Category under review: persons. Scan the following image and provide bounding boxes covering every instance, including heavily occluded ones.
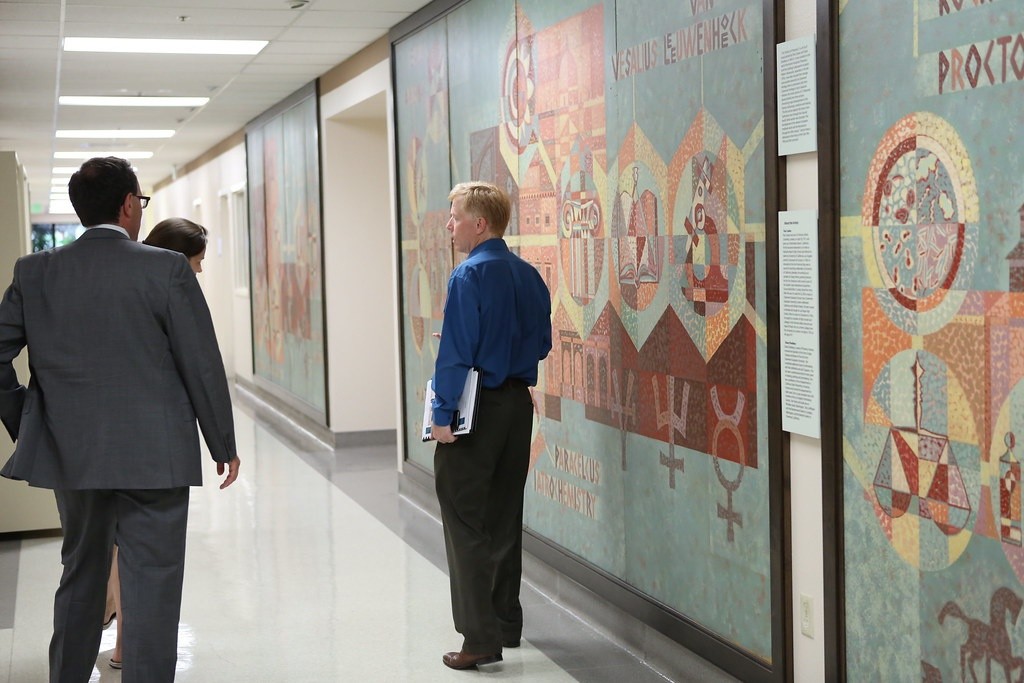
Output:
[431,180,553,668]
[0,156,241,683]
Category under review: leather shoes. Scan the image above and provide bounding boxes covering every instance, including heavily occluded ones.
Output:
[502,637,520,647]
[442,651,503,669]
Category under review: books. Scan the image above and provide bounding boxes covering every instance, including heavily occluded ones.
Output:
[422,367,484,442]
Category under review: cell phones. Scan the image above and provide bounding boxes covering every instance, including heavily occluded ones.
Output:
[450,410,460,432]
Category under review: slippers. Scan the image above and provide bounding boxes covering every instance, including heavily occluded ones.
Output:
[102,612,117,630]
[109,658,122,669]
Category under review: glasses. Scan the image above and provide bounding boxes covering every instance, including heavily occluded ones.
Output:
[121,196,150,208]
